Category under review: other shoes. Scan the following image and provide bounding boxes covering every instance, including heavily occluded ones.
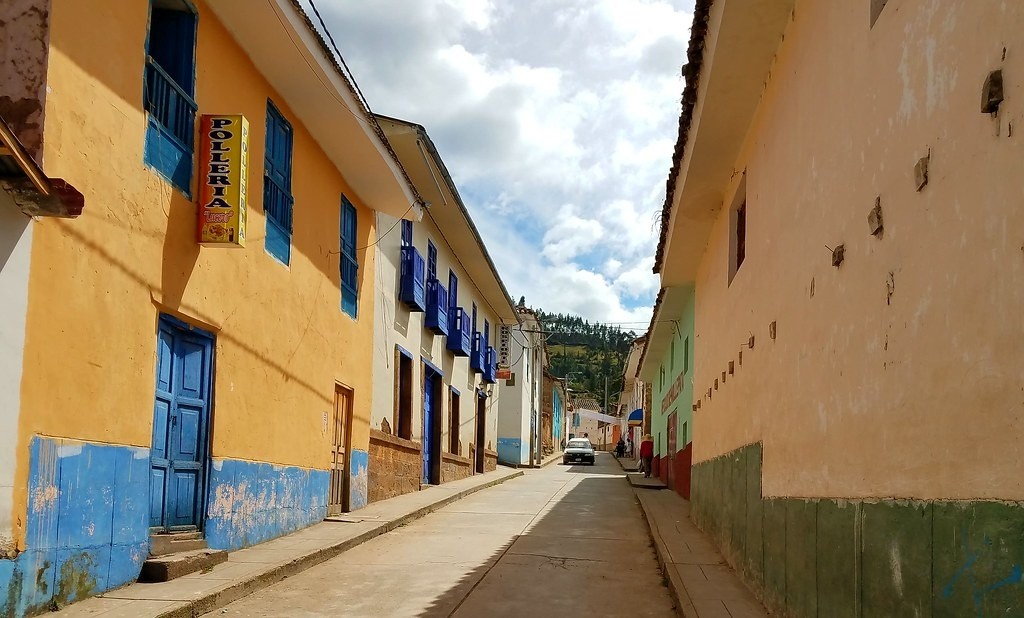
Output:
[619,457,621,458]
[644,473,650,478]
[623,456,624,458]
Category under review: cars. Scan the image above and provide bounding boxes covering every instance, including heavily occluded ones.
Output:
[562,438,596,466]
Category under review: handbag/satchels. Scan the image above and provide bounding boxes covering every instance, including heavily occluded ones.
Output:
[636,458,643,473]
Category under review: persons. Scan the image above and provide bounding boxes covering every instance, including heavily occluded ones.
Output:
[616,437,633,458]
[640,434,654,478]
[561,438,566,451]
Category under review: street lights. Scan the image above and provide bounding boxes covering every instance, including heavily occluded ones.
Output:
[564,371,583,448]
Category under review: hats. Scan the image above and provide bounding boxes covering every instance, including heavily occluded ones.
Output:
[641,434,652,441]
[584,433,588,436]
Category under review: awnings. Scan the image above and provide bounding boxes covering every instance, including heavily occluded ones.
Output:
[628,409,643,427]
